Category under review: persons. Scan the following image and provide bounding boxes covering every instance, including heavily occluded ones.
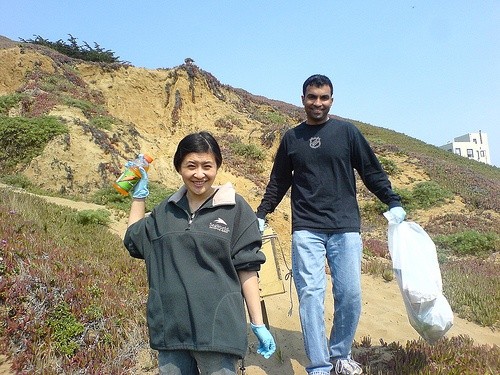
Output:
[124,131,275,375]
[254,74,407,375]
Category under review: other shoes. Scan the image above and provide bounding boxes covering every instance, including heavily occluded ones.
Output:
[330,357,363,375]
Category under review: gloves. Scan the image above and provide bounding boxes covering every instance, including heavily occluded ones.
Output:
[258,218,265,232]
[251,322,276,359]
[390,207,406,224]
[124,153,149,198]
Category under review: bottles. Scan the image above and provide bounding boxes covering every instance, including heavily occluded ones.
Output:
[113,154,154,196]
